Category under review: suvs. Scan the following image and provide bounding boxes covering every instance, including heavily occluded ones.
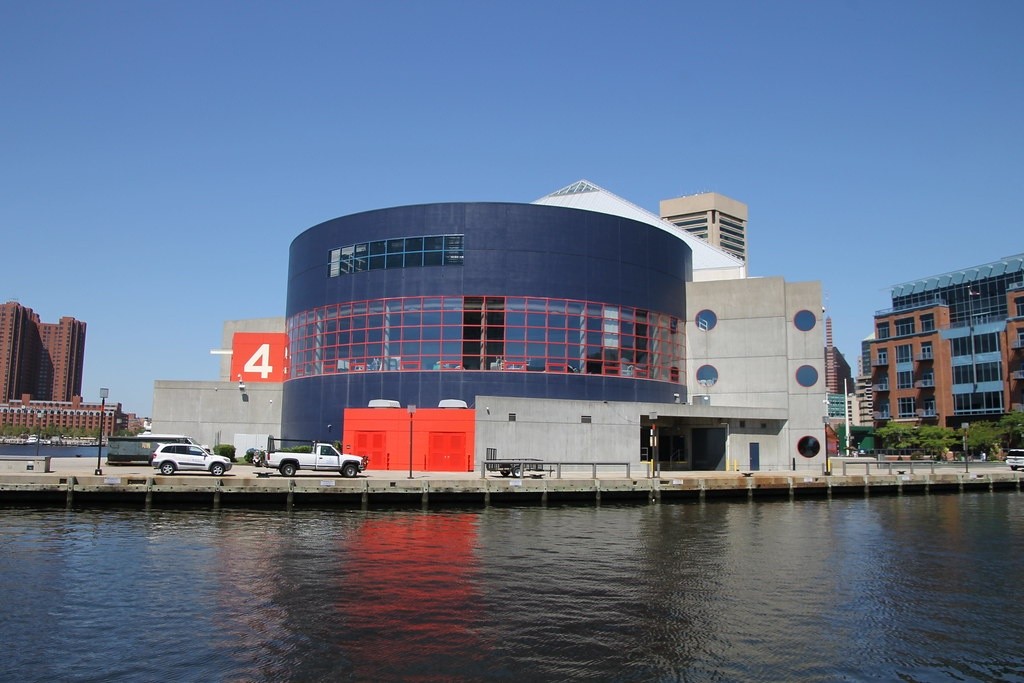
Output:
[1005,449,1024,470]
[151,442,233,476]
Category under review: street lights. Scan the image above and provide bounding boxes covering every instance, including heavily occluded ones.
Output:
[406,403,416,479]
[962,422,970,474]
[648,411,658,478]
[36,413,42,456]
[94,387,109,476]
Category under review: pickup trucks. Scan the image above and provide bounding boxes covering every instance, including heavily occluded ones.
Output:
[264,441,364,477]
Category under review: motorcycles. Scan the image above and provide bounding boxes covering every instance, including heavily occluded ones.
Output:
[252,448,262,467]
